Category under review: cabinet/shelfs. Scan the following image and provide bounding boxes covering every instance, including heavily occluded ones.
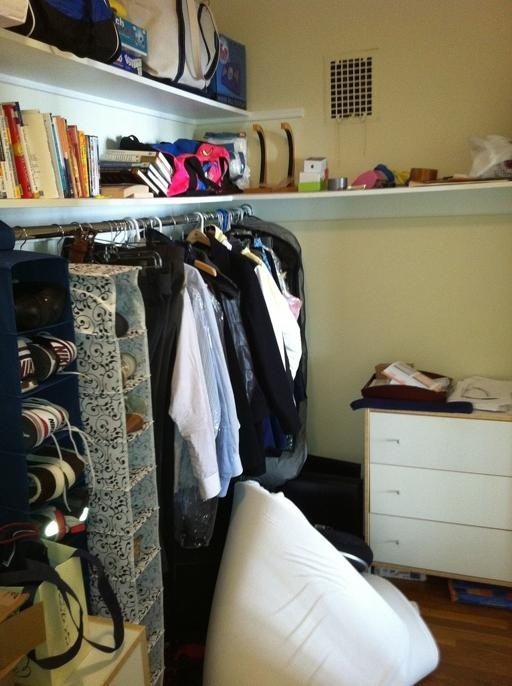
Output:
[66,260,167,686]
[0,218,94,611]
[361,406,512,595]
[0,23,247,209]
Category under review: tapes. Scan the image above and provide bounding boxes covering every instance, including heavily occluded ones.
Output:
[410,168,438,182]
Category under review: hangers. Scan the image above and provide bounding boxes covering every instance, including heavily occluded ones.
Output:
[13,204,251,275]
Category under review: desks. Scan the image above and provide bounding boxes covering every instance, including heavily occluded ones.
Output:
[57,613,152,686]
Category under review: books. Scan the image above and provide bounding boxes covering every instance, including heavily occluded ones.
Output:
[0,101,174,199]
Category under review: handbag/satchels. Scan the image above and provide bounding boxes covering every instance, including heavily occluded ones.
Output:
[1,542,126,684]
[121,135,243,196]
[17,0,122,66]
[122,0,222,94]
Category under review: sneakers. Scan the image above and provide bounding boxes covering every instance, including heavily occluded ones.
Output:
[117,314,146,435]
[14,273,89,542]
